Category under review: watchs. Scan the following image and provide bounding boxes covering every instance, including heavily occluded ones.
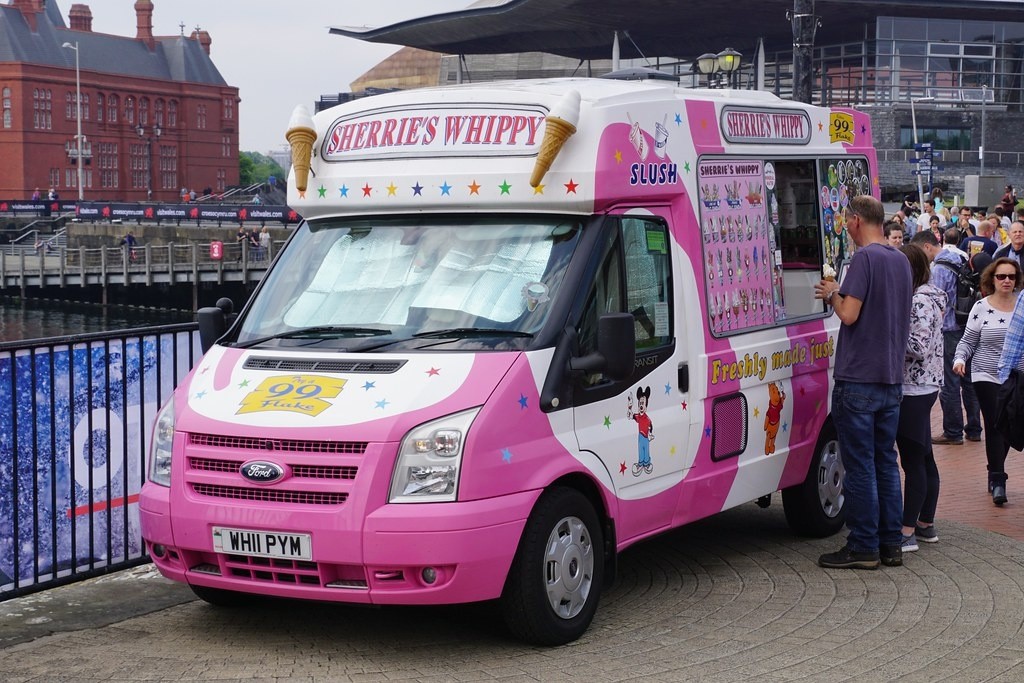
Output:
[827,289,840,304]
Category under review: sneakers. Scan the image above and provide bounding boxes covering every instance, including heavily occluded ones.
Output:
[878,546,903,566]
[900,533,919,553]
[913,524,938,542]
[819,541,881,569]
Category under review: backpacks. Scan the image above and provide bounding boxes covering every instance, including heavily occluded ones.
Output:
[935,260,978,325]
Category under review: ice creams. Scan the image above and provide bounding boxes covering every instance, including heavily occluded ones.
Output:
[530,89,582,188]
[285,104,318,190]
[704,214,772,331]
[823,267,837,282]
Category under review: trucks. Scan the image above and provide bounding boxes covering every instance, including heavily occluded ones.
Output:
[137,76,884,647]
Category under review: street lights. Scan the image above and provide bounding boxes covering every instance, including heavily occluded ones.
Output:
[697,48,719,89]
[717,47,744,89]
[61,41,94,199]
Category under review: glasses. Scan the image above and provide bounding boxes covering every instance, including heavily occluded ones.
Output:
[991,273,1017,281]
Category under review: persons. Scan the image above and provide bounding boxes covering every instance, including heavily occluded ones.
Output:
[814,196,912,569]
[953,258,1024,504]
[34,188,41,200]
[269,174,276,192]
[895,244,948,551]
[180,185,196,204]
[249,194,261,205]
[203,185,212,198]
[46,188,55,200]
[911,231,983,444]
[883,185,1024,382]
[237,226,270,263]
[34,238,44,253]
[119,231,137,265]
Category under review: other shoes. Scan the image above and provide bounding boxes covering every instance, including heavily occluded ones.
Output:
[929,433,963,445]
[965,432,981,441]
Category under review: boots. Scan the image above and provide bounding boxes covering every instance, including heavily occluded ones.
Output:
[985,465,1008,505]
